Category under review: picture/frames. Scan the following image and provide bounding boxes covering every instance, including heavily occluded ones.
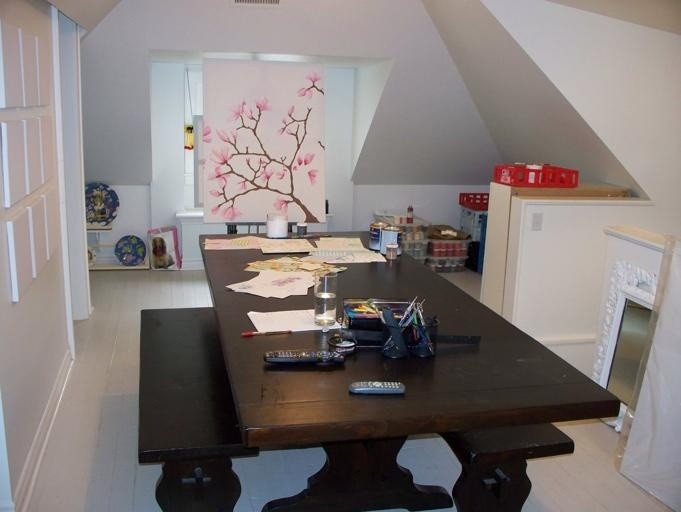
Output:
[596,286,655,434]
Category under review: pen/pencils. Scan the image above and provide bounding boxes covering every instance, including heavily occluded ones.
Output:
[345,295,435,355]
[241,330,292,337]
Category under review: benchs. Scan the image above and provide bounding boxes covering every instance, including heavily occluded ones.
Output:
[438,419,574,512]
[138,308,259,512]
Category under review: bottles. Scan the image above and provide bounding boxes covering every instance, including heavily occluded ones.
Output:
[297,222,308,236]
[385,243,399,261]
[407,205,413,224]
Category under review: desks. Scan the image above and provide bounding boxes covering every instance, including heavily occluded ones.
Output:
[198,230,620,512]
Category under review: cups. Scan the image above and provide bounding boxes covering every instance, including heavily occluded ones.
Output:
[265,205,288,239]
[524,164,543,186]
[314,269,337,327]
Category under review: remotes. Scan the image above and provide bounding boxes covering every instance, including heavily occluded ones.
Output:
[264,350,345,365]
[349,381,406,394]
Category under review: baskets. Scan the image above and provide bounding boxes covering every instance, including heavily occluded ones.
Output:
[492,161,581,189]
[458,190,490,210]
[373,211,489,273]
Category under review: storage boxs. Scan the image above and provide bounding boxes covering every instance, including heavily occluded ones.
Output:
[460,207,488,242]
